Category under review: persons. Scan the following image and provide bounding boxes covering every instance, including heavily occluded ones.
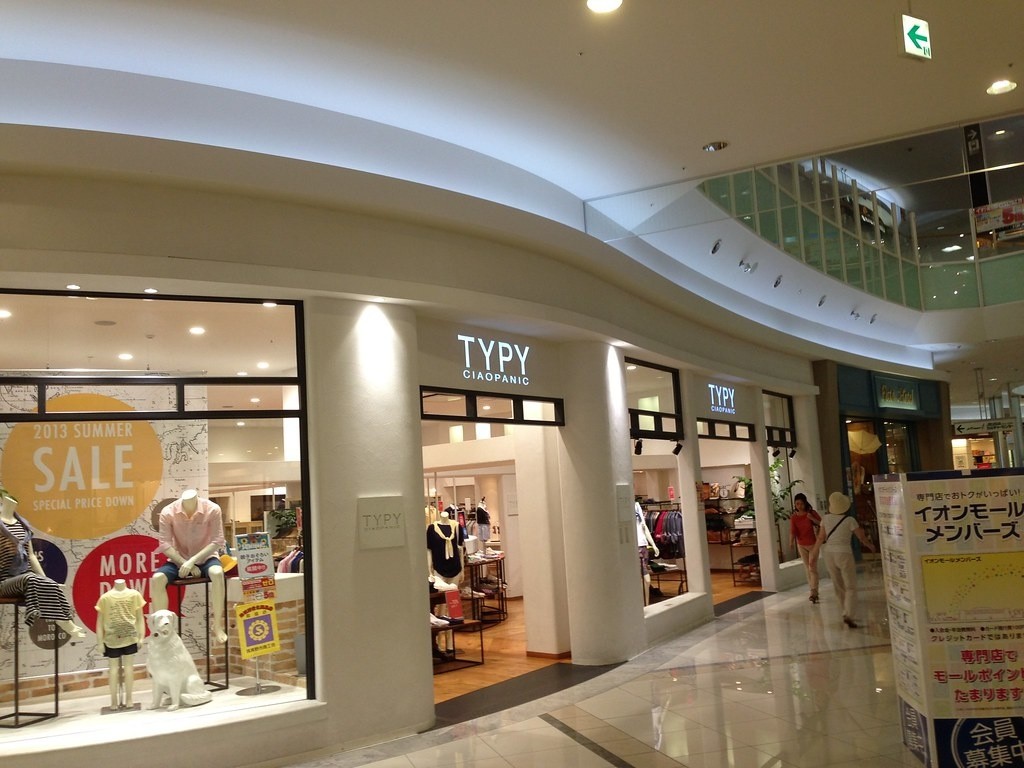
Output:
[477,501,491,554]
[808,492,876,628]
[635,501,660,606]
[94,579,147,710]
[0,496,87,642]
[790,493,821,604]
[152,489,228,643]
[426,512,464,654]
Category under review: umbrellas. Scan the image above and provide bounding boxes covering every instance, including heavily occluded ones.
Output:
[847,430,882,454]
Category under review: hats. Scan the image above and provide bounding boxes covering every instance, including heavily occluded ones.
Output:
[828,491,851,514]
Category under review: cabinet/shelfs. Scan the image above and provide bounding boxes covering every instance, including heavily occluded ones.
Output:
[704,498,748,545]
[455,560,509,633]
[730,545,761,587]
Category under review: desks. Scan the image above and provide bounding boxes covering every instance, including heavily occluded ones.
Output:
[169,574,230,693]
[0,597,59,728]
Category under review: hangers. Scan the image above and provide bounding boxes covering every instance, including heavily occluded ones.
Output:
[293,539,303,552]
[643,503,681,513]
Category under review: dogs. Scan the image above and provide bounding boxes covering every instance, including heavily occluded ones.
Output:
[143,609,213,711]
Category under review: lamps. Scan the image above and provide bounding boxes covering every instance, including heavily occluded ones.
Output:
[788,448,797,458]
[772,448,780,457]
[634,439,643,455]
[672,442,683,455]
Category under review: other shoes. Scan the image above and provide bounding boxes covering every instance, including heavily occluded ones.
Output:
[808,596,819,603]
[843,616,857,627]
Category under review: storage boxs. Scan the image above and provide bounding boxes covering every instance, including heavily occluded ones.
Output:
[734,519,754,529]
